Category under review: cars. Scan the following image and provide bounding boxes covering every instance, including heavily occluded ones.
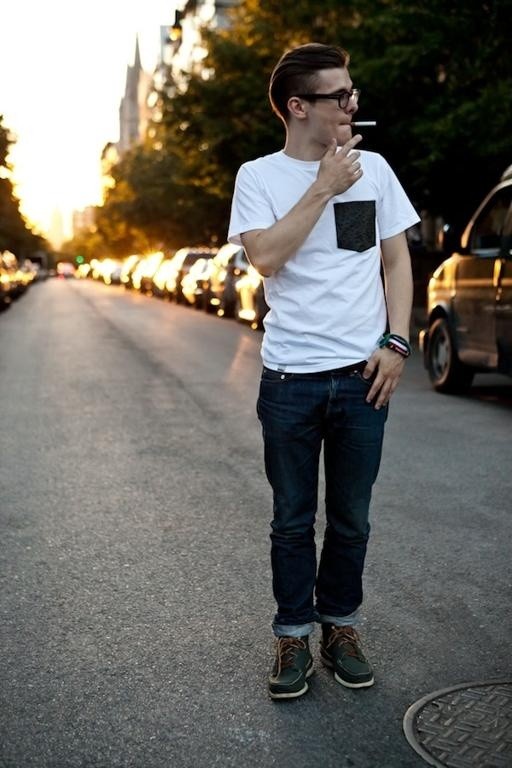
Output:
[84,244,270,329]
[0,251,39,313]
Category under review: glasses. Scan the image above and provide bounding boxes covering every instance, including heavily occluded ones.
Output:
[293,90,359,109]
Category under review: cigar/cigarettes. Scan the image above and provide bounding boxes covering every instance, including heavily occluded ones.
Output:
[347,121,378,127]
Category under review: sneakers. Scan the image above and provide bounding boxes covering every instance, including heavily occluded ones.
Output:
[320,623,374,688]
[268,637,315,698]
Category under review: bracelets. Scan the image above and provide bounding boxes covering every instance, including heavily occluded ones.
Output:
[380,333,412,359]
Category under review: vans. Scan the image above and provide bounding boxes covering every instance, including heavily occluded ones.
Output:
[419,165,512,391]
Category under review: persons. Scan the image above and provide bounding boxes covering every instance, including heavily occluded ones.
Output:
[227,42,421,702]
